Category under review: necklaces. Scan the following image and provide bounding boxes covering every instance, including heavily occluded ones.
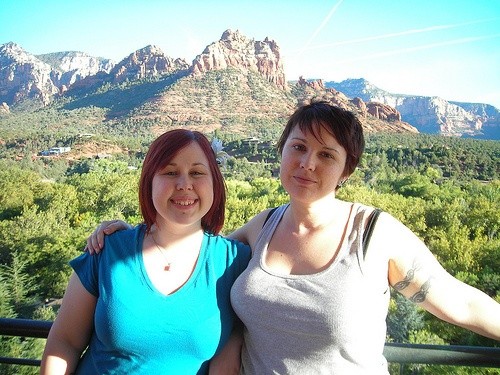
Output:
[150,233,172,271]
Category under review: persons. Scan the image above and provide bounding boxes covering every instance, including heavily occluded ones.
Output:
[82,99,500,375]
[41,129,252,375]
